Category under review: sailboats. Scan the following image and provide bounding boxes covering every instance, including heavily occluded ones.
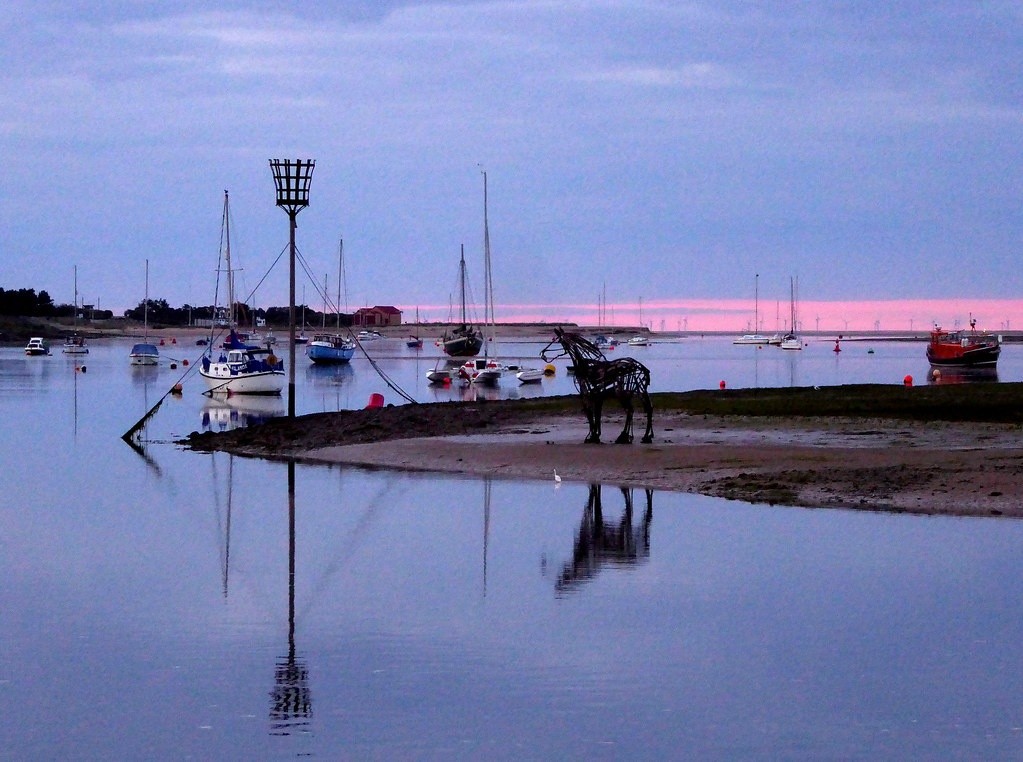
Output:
[406,306,423,348]
[442,243,484,356]
[129,258,158,366]
[591,281,650,349]
[199,189,286,394]
[733,274,803,350]
[63,265,88,353]
[295,239,356,363]
[425,171,502,384]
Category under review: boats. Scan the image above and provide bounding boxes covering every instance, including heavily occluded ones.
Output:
[435,342,444,347]
[516,365,545,382]
[565,360,637,379]
[925,312,1002,367]
[358,329,382,341]
[24,337,50,355]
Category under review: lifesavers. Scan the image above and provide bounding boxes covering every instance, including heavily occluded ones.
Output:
[466,337,475,346]
[267,354,277,365]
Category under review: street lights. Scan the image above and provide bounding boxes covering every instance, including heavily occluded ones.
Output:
[267,158,317,419]
